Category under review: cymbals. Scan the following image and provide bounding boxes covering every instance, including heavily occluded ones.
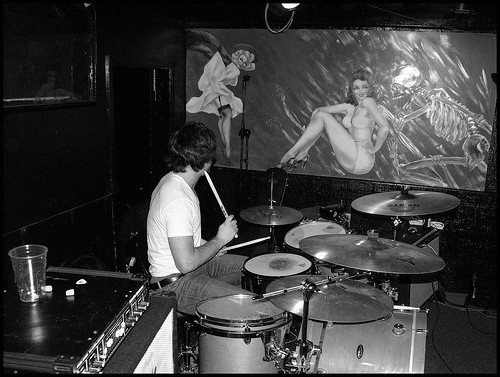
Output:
[266,273,394,327]
[239,205,304,226]
[351,187,462,216]
[298,232,446,275]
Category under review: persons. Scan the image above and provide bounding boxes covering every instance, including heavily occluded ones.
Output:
[149,120,256,314]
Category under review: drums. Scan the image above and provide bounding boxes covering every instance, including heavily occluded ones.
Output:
[241,252,314,295]
[295,309,428,374]
[194,291,294,374]
[283,219,347,259]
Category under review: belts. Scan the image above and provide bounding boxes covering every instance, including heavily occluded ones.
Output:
[149,274,182,290]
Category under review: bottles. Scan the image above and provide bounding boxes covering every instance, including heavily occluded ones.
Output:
[469,272,480,303]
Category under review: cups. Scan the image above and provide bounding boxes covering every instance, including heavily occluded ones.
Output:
[9,245,48,302]
